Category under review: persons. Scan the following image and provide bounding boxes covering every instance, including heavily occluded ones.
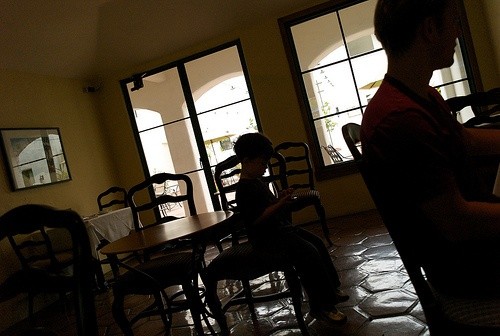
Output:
[234,134,350,326]
[362,0,500,336]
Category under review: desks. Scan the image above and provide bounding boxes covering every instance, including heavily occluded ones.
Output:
[99,209,235,336]
[32,207,143,293]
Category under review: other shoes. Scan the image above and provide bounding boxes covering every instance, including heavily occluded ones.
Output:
[309,307,348,324]
[329,292,350,305]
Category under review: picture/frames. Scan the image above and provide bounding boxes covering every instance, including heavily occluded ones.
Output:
[0,126,73,193]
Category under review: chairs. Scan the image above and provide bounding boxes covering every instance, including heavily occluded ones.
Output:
[201,151,312,336]
[0,204,98,336]
[445,87,500,130]
[7,226,101,322]
[95,186,127,285]
[267,140,334,248]
[342,123,500,336]
[128,172,207,327]
